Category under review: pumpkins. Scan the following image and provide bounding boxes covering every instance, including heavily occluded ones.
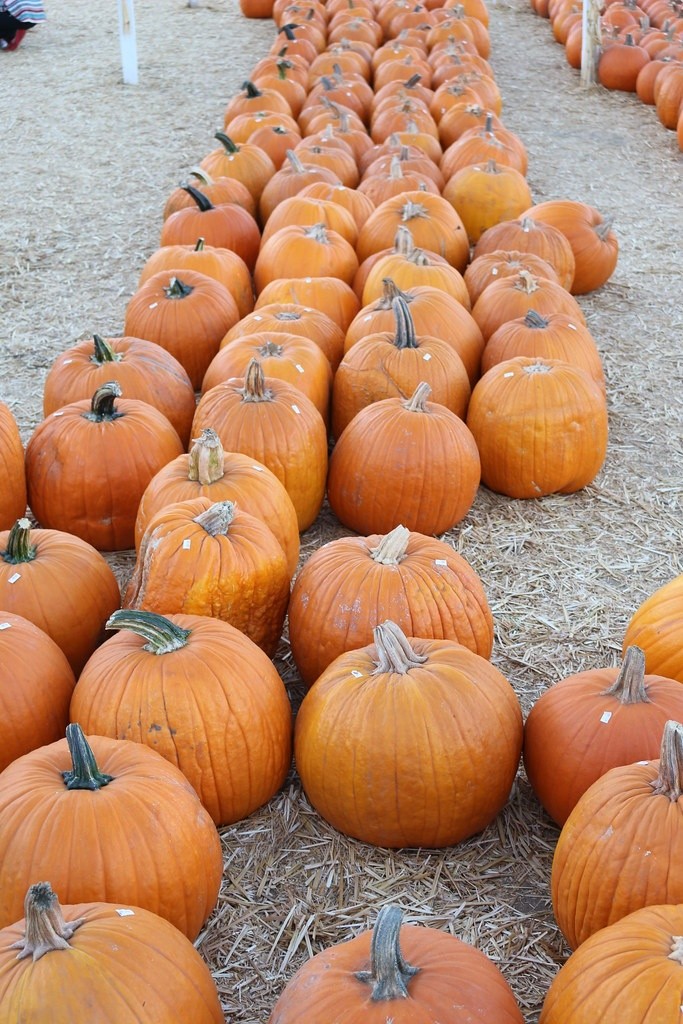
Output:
[0,0,683,1024]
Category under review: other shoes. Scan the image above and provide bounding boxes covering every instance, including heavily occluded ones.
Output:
[5,28,27,51]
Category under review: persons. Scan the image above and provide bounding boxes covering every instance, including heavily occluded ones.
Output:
[0,0,49,50]
